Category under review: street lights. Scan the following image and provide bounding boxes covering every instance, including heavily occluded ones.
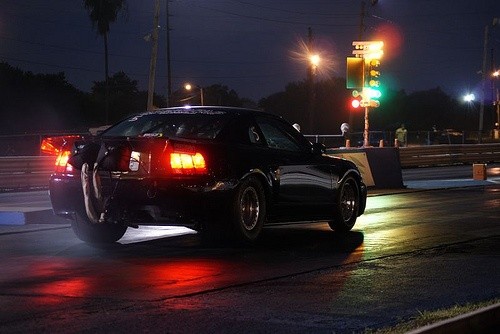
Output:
[305,27,322,144]
[186,84,204,106]
[351,41,383,147]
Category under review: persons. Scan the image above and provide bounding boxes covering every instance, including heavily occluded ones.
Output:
[429,125,439,143]
[395,124,408,147]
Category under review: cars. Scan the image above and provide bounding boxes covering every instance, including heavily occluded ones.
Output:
[48,105,368,249]
[441,128,463,139]
